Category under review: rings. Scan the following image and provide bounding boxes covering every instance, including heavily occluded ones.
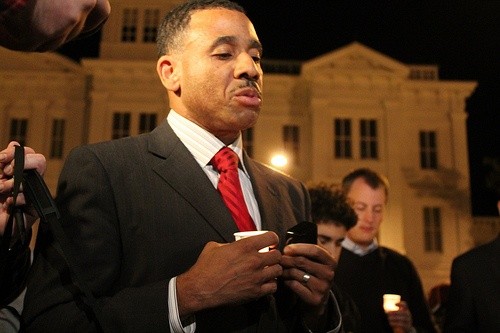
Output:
[300,272,311,284]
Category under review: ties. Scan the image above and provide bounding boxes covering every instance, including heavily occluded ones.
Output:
[207,147,257,232]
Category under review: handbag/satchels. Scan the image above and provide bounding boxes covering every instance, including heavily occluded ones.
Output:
[0,146,32,308]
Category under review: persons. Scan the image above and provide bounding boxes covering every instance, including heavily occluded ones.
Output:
[0,0,111,52]
[307,168,500,333]
[19,0,341,333]
[0,141,47,309]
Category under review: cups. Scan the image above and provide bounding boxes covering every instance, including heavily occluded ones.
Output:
[233,229,271,284]
[382,291,401,313]
